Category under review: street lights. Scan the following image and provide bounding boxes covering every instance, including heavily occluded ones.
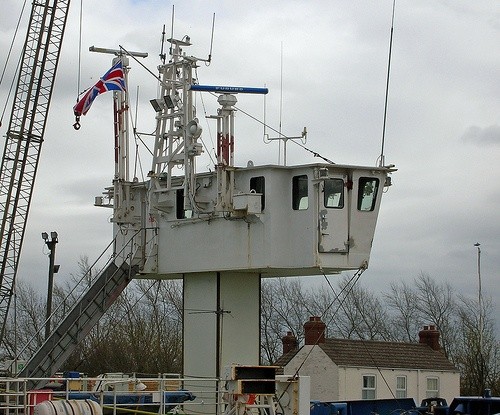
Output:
[41,231,60,340]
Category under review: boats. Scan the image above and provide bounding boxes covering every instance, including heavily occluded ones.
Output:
[0,0,500,415]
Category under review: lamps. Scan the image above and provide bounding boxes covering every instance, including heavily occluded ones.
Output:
[150,98,164,112]
[164,93,181,109]
[136,380,147,391]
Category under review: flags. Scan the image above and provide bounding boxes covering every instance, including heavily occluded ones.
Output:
[73,59,126,116]
